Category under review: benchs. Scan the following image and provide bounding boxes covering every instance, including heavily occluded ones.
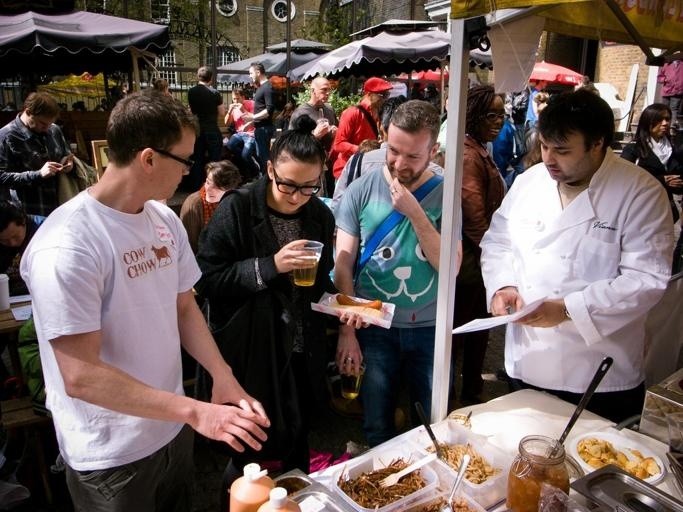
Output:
[1,372,201,503]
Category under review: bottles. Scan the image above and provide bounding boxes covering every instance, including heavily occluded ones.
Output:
[255,486,300,511]
[229,462,274,511]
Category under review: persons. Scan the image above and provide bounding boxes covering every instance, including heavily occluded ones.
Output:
[192,131,370,480]
[447,85,507,412]
[0,60,683,295]
[20,93,270,511]
[480,86,675,427]
[336,101,462,447]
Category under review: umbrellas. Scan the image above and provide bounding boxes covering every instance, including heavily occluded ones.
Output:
[210,37,333,87]
[286,18,493,115]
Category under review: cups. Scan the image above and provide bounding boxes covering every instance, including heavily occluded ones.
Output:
[292,240,325,288]
[338,360,367,400]
[0,274,11,311]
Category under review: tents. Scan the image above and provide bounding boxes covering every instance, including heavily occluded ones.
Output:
[0,11,177,93]
[432,0,683,421]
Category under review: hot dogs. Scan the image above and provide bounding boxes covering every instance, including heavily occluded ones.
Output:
[329,294,384,320]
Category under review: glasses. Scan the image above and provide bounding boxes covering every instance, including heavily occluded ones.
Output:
[273,167,321,196]
[378,93,390,97]
[487,112,510,120]
[152,148,194,171]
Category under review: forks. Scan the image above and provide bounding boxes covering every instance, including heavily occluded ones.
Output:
[377,451,437,489]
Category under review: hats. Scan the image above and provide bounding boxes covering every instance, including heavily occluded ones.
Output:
[364,77,393,92]
